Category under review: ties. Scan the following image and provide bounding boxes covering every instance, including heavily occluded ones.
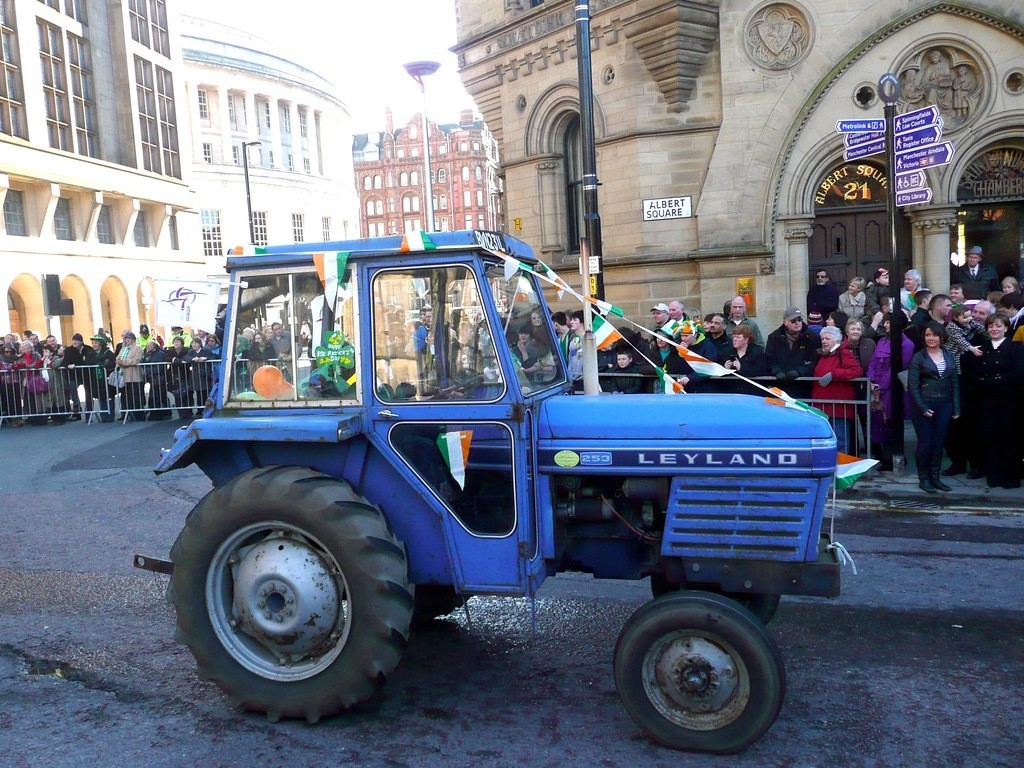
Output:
[972,269,975,277]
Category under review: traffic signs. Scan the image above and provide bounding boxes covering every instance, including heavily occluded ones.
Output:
[894,124,942,153]
[894,140,955,175]
[895,186,933,206]
[893,105,944,136]
[835,118,887,133]
[843,136,886,163]
[894,168,927,194]
[842,130,886,149]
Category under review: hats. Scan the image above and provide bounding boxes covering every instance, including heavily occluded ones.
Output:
[874,269,889,279]
[650,302,669,314]
[653,318,680,340]
[966,246,985,258]
[124,333,135,340]
[783,307,804,322]
[72,333,83,341]
[807,313,822,326]
[90,334,106,344]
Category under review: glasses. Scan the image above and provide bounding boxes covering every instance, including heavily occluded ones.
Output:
[4,350,13,354]
[787,317,804,324]
[815,274,826,279]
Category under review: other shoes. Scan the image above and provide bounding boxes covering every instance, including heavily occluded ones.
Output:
[878,460,909,471]
[967,469,979,478]
[12,411,204,427]
[942,460,967,475]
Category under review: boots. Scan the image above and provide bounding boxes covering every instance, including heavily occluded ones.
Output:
[931,449,951,492]
[916,452,938,493]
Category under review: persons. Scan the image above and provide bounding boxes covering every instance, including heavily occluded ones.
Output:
[232,322,303,397]
[0,322,221,428]
[950,246,1001,300]
[765,267,1024,494]
[597,293,768,397]
[376,302,584,402]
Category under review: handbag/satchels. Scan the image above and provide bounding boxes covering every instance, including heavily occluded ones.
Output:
[108,371,125,388]
[28,373,49,394]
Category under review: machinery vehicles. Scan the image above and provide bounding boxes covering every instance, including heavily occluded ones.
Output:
[133,227,847,757]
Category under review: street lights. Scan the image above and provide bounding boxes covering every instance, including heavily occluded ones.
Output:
[405,59,444,232]
[243,141,263,334]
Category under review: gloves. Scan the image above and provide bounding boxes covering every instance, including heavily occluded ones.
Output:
[819,372,835,388]
[775,372,789,389]
[789,369,799,379]
